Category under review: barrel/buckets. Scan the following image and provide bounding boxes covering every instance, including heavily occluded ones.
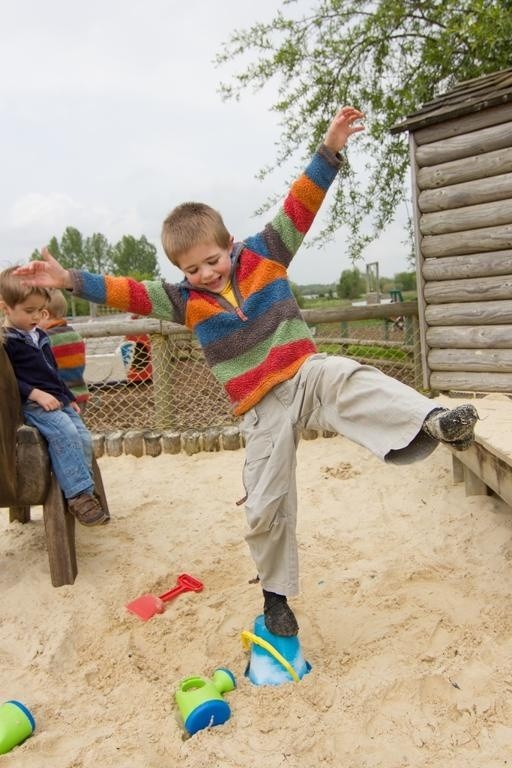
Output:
[241,613,312,686]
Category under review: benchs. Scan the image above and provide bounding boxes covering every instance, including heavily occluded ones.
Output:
[449,393,512,510]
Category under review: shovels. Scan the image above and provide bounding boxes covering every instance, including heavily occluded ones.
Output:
[126,574,203,622]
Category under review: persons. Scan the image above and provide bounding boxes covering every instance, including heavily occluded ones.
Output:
[38,286,89,417]
[0,267,109,528]
[12,107,477,639]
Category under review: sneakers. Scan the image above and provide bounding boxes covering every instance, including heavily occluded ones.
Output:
[68,492,106,526]
